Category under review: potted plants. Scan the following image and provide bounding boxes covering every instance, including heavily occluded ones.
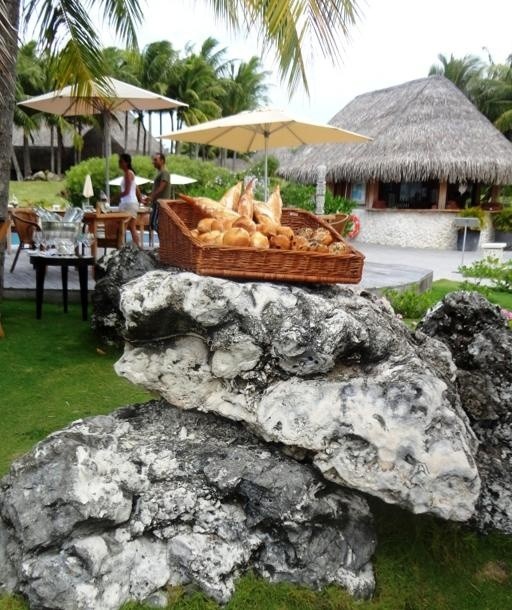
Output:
[454,204,512,251]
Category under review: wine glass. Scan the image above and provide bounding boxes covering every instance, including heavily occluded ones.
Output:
[33,231,95,259]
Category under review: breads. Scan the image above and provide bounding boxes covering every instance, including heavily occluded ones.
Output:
[176,179,352,254]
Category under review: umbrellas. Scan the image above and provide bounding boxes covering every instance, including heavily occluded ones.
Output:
[12,73,189,203]
[159,105,375,198]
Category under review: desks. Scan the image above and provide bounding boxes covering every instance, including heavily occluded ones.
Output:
[51,207,155,281]
[481,242,507,261]
[27,250,95,322]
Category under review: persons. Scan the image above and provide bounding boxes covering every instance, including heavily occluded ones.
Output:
[147,153,171,249]
[118,153,142,249]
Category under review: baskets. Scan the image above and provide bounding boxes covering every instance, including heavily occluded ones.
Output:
[157,196,362,284]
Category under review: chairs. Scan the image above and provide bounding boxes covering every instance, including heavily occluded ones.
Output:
[8,207,42,274]
[324,213,348,236]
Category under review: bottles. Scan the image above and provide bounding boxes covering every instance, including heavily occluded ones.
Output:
[100,191,107,206]
